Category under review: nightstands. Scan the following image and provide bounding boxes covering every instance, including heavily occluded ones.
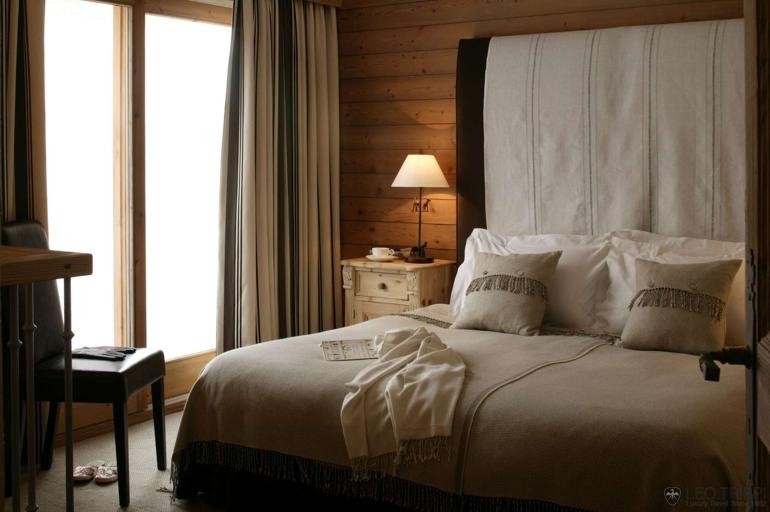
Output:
[339,256,457,327]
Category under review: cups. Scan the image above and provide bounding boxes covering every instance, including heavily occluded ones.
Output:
[372,248,394,257]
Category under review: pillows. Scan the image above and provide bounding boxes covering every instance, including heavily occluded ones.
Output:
[607,229,746,348]
[447,251,564,335]
[613,258,743,355]
[451,228,612,336]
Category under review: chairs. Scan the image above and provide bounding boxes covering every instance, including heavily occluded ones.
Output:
[3,218,166,510]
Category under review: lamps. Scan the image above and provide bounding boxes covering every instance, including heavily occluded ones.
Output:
[391,153,450,264]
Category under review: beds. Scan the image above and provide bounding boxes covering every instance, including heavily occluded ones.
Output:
[170,16,748,512]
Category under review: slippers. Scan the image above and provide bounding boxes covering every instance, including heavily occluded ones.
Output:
[72,461,118,483]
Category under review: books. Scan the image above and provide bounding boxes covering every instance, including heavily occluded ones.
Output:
[318,339,379,362]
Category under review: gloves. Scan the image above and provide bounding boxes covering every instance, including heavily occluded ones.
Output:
[72,345,137,361]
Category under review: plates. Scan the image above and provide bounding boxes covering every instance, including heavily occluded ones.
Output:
[366,255,394,262]
